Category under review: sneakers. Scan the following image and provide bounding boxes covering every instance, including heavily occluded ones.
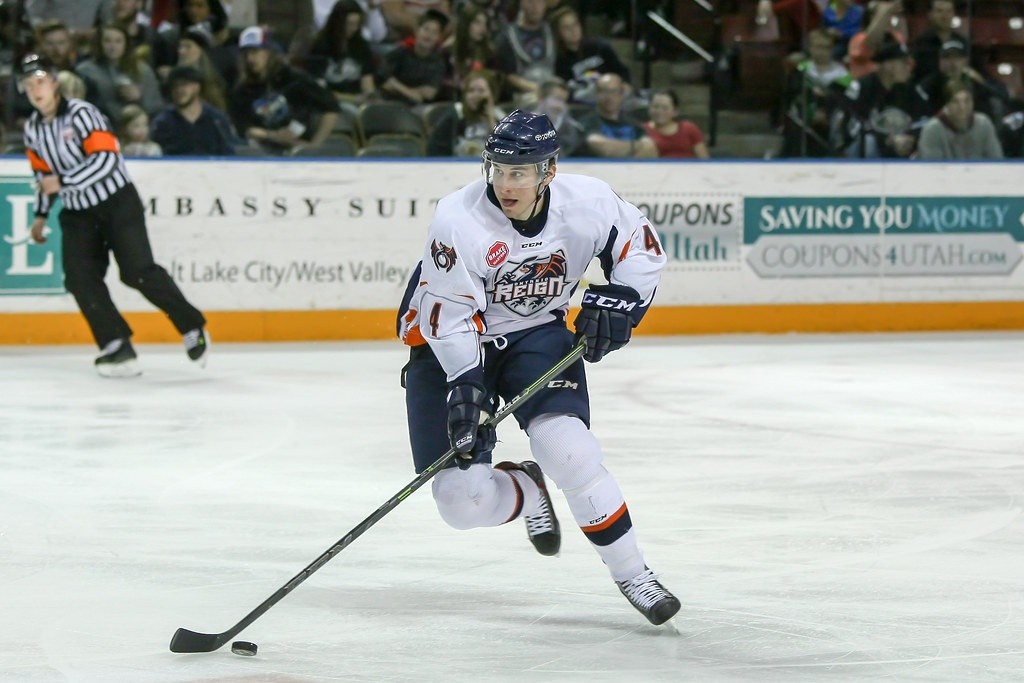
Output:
[494,461,561,558]
[95,337,142,378]
[602,558,681,636]
[184,329,210,369]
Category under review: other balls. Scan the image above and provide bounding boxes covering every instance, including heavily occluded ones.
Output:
[231,640,258,657]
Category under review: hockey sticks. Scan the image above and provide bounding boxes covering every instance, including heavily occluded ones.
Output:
[166,342,591,656]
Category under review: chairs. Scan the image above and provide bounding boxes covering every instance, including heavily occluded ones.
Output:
[286,101,457,162]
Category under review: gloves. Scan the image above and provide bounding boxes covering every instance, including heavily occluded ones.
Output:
[447,381,496,470]
[573,284,640,363]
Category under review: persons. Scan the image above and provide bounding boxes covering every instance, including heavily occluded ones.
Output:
[719,0,1024,158]
[0,0,713,159]
[15,55,212,378]
[396,110,684,635]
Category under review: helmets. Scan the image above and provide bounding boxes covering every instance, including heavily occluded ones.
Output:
[17,53,52,81]
[485,110,559,165]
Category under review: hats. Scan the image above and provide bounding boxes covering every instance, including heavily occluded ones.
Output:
[939,40,967,58]
[239,26,272,52]
[168,66,202,81]
[869,41,911,62]
[180,29,212,55]
